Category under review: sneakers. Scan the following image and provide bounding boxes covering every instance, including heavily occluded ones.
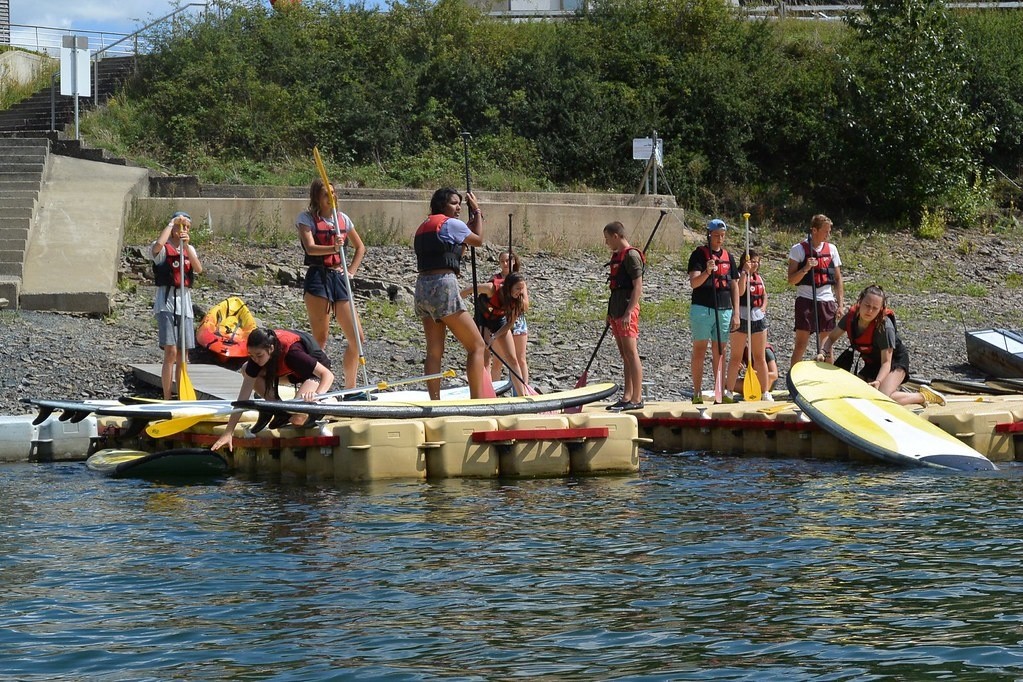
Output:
[725,390,733,399]
[692,396,703,403]
[919,384,947,409]
[761,391,774,402]
[606,399,644,410]
[714,396,734,403]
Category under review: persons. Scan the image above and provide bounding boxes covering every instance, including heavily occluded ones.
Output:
[788,215,845,396]
[725,251,774,401]
[603,221,646,410]
[726,335,779,394]
[294,178,378,401]
[810,284,947,407]
[414,188,485,401]
[458,272,524,398]
[489,252,529,396]
[686,218,740,405]
[210,327,335,452]
[150,212,202,400]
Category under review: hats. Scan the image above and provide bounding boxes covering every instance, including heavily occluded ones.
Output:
[172,212,192,222]
[708,219,726,231]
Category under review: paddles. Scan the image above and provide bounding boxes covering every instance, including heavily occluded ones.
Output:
[146,369,456,438]
[177,222,196,400]
[706,227,723,404]
[562,209,668,413]
[807,205,820,355]
[403,286,539,396]
[756,397,995,414]
[508,213,517,397]
[459,131,496,398]
[743,213,762,402]
[312,146,371,402]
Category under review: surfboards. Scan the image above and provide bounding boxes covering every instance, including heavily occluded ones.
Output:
[787,358,1002,474]
[84,447,229,477]
[18,379,515,427]
[231,380,619,417]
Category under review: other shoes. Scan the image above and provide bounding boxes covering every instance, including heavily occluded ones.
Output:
[269,412,292,429]
[303,413,325,429]
[343,392,378,401]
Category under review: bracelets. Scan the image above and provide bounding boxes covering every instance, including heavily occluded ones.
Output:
[332,246,336,254]
[472,207,483,217]
[800,267,808,274]
[819,349,828,357]
[705,269,710,276]
